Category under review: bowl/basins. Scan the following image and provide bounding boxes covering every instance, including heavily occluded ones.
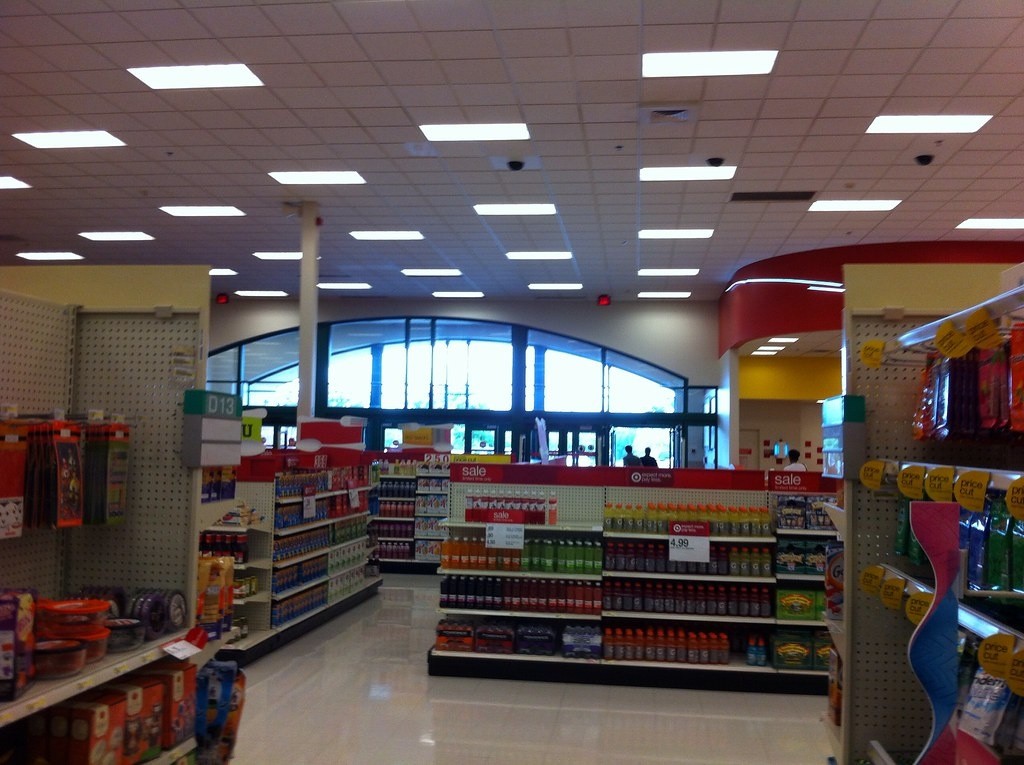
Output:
[30,597,145,679]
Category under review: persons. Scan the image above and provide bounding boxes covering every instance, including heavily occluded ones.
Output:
[783,449,808,472]
[639,447,658,466]
[623,446,643,467]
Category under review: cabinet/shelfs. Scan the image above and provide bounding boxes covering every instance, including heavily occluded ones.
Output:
[426,465,839,695]
[819,283,1024,765]
[202,464,390,671]
[372,456,453,576]
[0,260,213,765]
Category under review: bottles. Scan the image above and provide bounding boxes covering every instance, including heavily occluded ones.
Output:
[370,458,379,485]
[746,638,767,665]
[438,576,601,616]
[464,489,546,526]
[379,523,413,538]
[227,616,248,645]
[380,459,383,475]
[378,501,414,518]
[549,491,557,526]
[602,625,730,666]
[436,619,600,660]
[394,459,416,476]
[197,533,249,565]
[440,534,602,575]
[384,458,389,475]
[604,581,773,619]
[602,500,771,539]
[603,541,772,578]
[383,482,416,498]
[233,575,257,599]
[375,542,410,559]
[377,481,381,497]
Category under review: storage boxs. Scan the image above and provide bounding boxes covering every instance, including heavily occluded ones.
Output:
[827,547,843,621]
[828,647,843,727]
[22,661,201,765]
[412,477,448,561]
